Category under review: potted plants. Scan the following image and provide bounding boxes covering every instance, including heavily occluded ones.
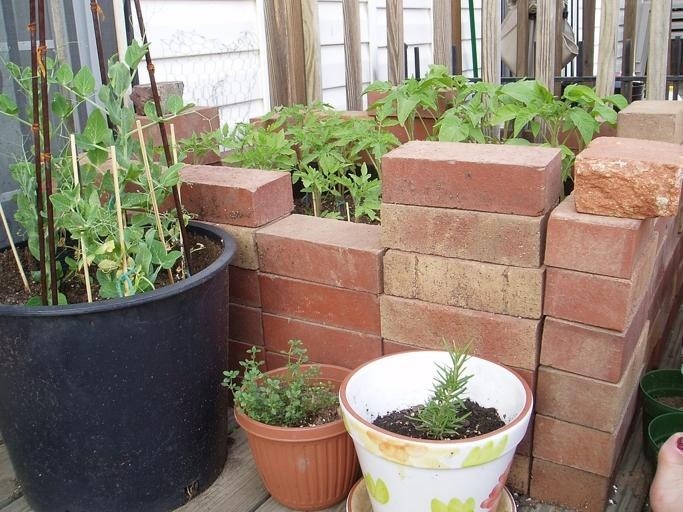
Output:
[0,16,238,512]
